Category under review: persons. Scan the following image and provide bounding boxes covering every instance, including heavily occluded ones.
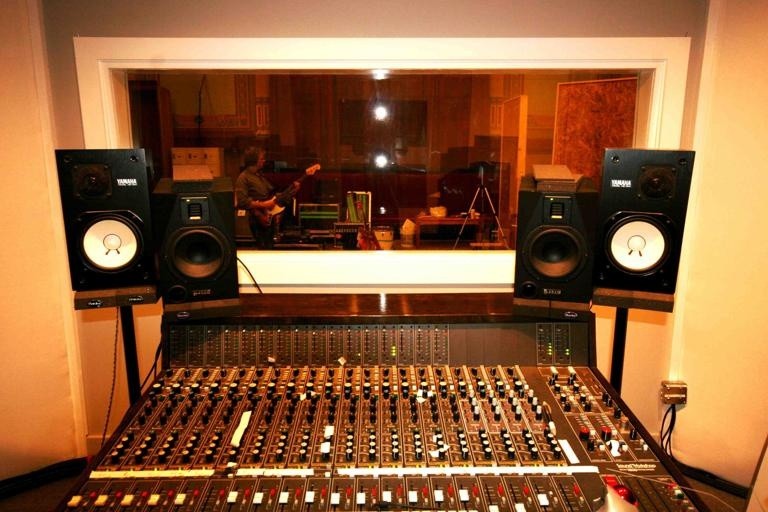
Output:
[234,145,300,250]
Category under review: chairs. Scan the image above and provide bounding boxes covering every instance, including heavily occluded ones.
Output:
[333,190,372,251]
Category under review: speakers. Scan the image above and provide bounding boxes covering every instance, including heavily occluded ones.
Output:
[150,177,240,304]
[512,176,601,304]
[54,148,153,292]
[595,147,695,295]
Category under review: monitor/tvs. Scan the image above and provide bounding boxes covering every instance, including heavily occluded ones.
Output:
[337,98,428,147]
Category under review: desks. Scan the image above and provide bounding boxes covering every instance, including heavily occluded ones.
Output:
[416,213,493,245]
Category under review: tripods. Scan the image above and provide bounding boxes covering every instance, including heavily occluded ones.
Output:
[452,168,509,250]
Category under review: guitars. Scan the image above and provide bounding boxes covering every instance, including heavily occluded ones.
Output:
[252,163,321,226]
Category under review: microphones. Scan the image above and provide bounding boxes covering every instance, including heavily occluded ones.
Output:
[264,160,287,170]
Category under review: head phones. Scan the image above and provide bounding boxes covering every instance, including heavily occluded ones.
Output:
[244,147,258,166]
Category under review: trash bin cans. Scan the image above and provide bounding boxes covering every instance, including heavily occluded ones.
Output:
[400,219,416,248]
